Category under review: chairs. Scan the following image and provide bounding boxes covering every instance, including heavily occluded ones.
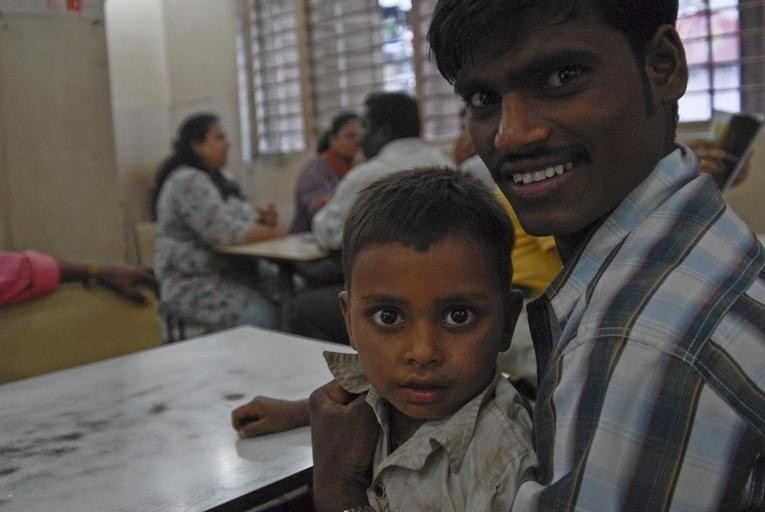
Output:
[133,220,189,343]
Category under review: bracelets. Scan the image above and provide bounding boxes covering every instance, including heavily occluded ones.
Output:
[79,260,98,291]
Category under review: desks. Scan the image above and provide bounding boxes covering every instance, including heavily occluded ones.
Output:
[217,233,344,334]
[0,323,368,512]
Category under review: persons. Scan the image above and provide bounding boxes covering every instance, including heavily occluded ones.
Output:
[146,111,282,335]
[231,167,543,512]
[293,88,563,297]
[308,0,764,512]
[0,250,159,308]
